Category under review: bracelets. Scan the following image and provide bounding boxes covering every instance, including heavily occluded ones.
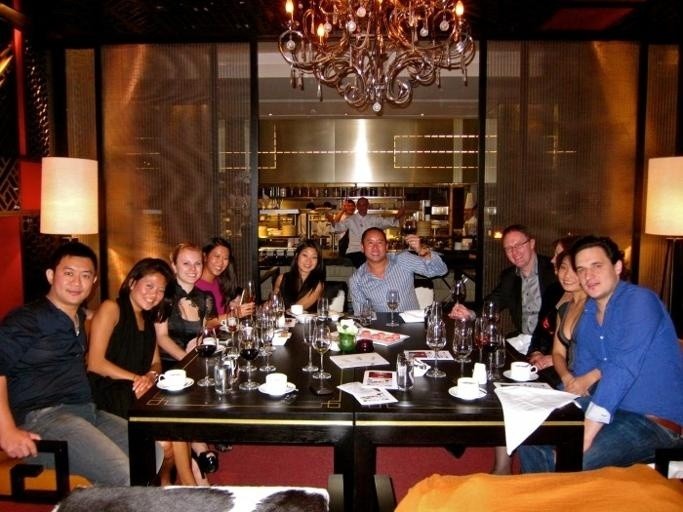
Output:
[419,251,429,257]
[131,373,137,381]
[144,371,159,383]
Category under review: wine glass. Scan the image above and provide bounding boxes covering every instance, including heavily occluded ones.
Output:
[196,279,507,394]
[402,215,418,254]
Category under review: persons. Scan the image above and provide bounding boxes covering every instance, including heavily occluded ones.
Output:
[444,224,558,460]
[153,241,218,486]
[490,237,579,477]
[348,227,448,316]
[335,200,355,251]
[555,250,592,400]
[0,244,164,486]
[194,237,255,452]
[519,236,682,472]
[273,240,325,311]
[85,256,198,485]
[325,198,406,259]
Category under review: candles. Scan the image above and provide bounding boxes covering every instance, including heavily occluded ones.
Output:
[455,1,464,24]
[317,23,324,44]
[286,1,294,20]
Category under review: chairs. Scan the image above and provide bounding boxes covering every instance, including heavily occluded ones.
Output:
[324,280,348,312]
[1,438,93,506]
[646,338,682,478]
[414,279,435,306]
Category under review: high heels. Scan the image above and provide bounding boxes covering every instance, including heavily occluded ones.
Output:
[197,451,218,478]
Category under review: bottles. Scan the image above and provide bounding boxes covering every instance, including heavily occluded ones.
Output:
[260,186,402,198]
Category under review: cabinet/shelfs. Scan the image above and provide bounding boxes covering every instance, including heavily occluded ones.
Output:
[258,186,451,249]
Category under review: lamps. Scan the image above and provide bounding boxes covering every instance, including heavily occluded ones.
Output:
[646,157,683,312]
[277,0,476,113]
[39,157,100,241]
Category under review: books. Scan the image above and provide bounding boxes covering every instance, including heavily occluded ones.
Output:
[362,369,399,391]
[329,352,390,369]
[404,350,455,362]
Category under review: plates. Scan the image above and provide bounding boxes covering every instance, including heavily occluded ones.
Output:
[259,224,298,238]
[155,304,540,400]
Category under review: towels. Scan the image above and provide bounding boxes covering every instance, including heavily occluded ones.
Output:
[493,386,583,458]
[398,309,427,324]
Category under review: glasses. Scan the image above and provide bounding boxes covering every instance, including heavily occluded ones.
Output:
[504,239,530,251]
